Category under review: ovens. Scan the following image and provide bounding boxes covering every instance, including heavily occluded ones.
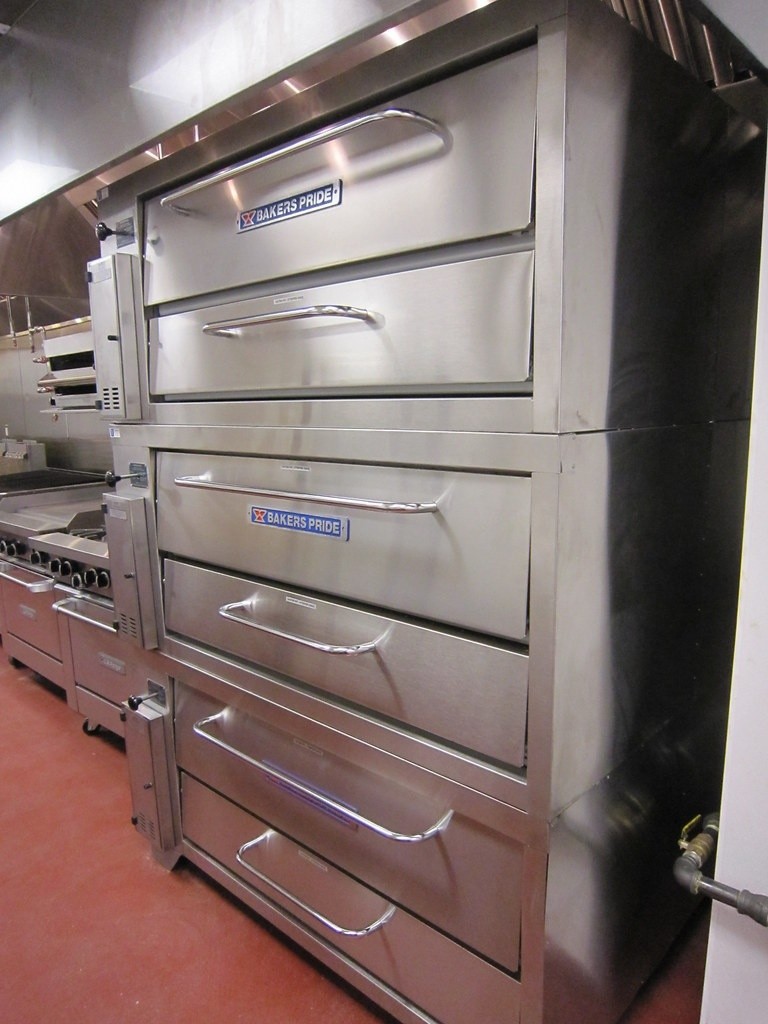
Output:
[86,0,768,1024]
[39,330,96,417]
[0,514,128,739]
